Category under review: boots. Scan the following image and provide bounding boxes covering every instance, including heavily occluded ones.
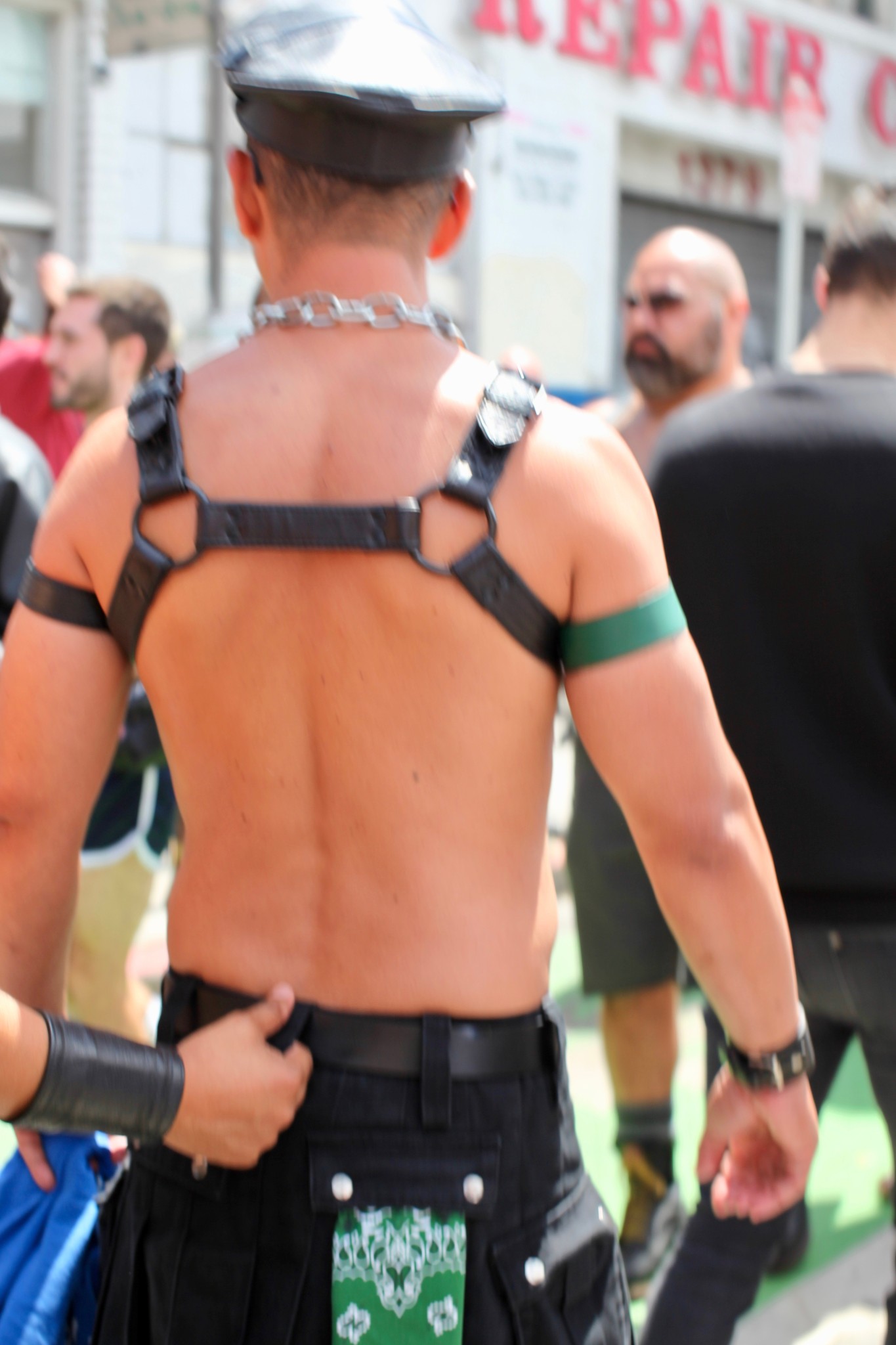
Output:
[611,1137,687,1297]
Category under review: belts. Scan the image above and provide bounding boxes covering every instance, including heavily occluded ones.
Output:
[162,966,540,1081]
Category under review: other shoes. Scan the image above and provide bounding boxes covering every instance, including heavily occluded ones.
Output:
[768,1198,810,1275]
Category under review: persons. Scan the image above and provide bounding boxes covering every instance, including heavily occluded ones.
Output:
[569,223,811,1305]
[0,0,821,1344]
[0,255,184,1047]
[0,983,311,1166]
[643,183,896,1343]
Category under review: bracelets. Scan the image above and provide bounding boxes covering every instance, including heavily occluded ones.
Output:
[724,1000,816,1089]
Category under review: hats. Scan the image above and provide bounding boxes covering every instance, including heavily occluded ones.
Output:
[222,0,505,182]
